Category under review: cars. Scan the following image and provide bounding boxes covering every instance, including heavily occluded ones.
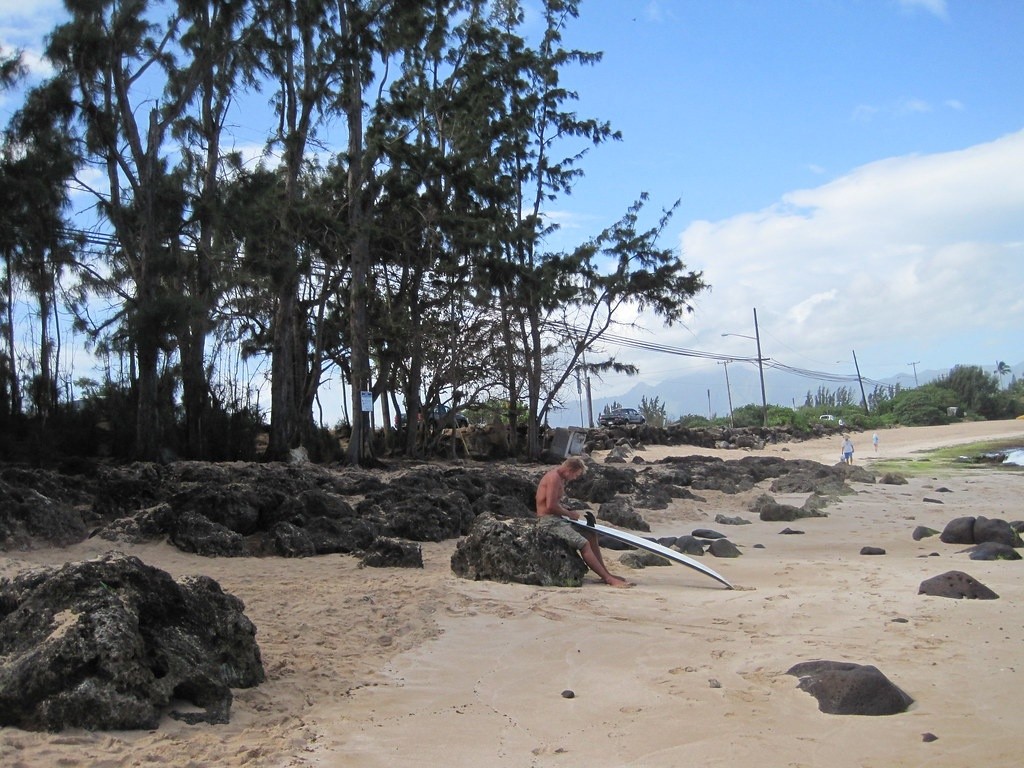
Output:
[820,414,834,421]
[395,403,470,430]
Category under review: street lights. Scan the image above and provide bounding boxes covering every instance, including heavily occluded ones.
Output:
[721,333,768,427]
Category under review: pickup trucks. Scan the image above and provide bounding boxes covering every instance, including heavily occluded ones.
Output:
[599,407,645,428]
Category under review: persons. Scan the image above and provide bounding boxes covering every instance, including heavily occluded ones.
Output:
[535,457,630,588]
[841,435,854,466]
[873,434,879,453]
[838,418,844,426]
